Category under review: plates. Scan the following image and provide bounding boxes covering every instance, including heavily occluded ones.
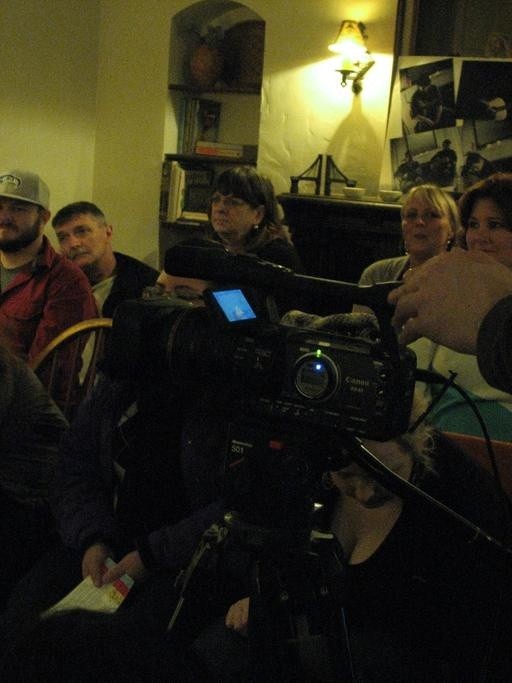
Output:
[220,20,263,88]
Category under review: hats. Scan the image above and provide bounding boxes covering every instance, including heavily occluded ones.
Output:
[0,169,50,210]
[165,239,226,281]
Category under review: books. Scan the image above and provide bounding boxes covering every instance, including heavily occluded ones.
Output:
[160,97,258,227]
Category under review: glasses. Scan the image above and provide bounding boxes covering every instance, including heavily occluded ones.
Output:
[141,287,204,304]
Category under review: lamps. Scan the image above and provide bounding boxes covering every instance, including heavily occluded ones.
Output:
[327,16,379,95]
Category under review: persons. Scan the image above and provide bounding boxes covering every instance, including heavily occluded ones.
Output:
[0,239,251,683]
[386,249,512,395]
[0,316,69,611]
[394,74,498,194]
[407,173,512,444]
[351,185,465,327]
[52,202,160,319]
[157,166,321,329]
[0,169,99,407]
[186,389,512,683]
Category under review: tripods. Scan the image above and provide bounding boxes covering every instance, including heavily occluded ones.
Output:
[166,430,512,683]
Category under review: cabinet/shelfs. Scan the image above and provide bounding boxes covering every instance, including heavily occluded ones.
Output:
[160,80,262,235]
[277,190,404,282]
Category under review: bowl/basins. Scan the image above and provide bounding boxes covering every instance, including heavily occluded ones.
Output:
[378,188,402,203]
[342,187,367,200]
